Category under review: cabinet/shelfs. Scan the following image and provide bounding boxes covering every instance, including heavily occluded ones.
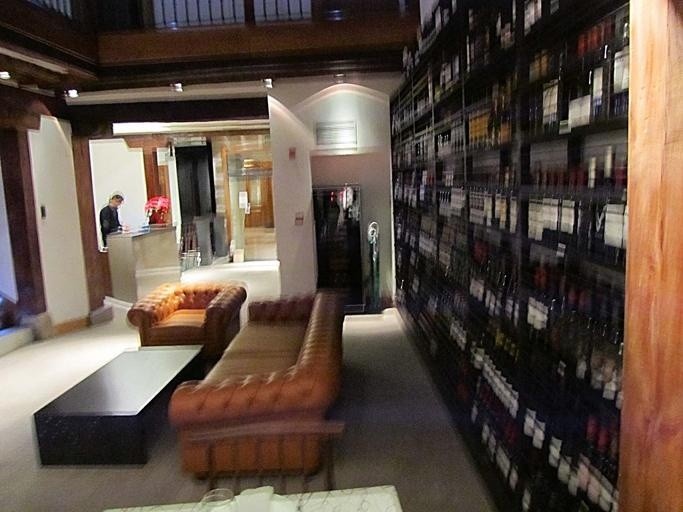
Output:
[436,2,516,509]
[512,1,682,510]
[388,57,436,389]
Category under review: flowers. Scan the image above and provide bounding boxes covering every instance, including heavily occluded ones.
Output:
[141,194,170,219]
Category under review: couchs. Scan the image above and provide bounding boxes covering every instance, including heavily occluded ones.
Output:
[125,280,248,365]
[166,289,346,484]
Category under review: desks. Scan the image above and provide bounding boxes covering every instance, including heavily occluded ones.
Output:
[100,484,407,512]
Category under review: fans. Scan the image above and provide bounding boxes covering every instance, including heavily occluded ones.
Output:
[366,221,381,314]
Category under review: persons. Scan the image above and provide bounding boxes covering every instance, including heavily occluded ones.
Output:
[98,193,124,249]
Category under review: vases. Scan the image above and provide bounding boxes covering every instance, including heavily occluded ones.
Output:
[152,210,165,223]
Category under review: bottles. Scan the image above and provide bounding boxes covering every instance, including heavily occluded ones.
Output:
[392,0,627,511]
